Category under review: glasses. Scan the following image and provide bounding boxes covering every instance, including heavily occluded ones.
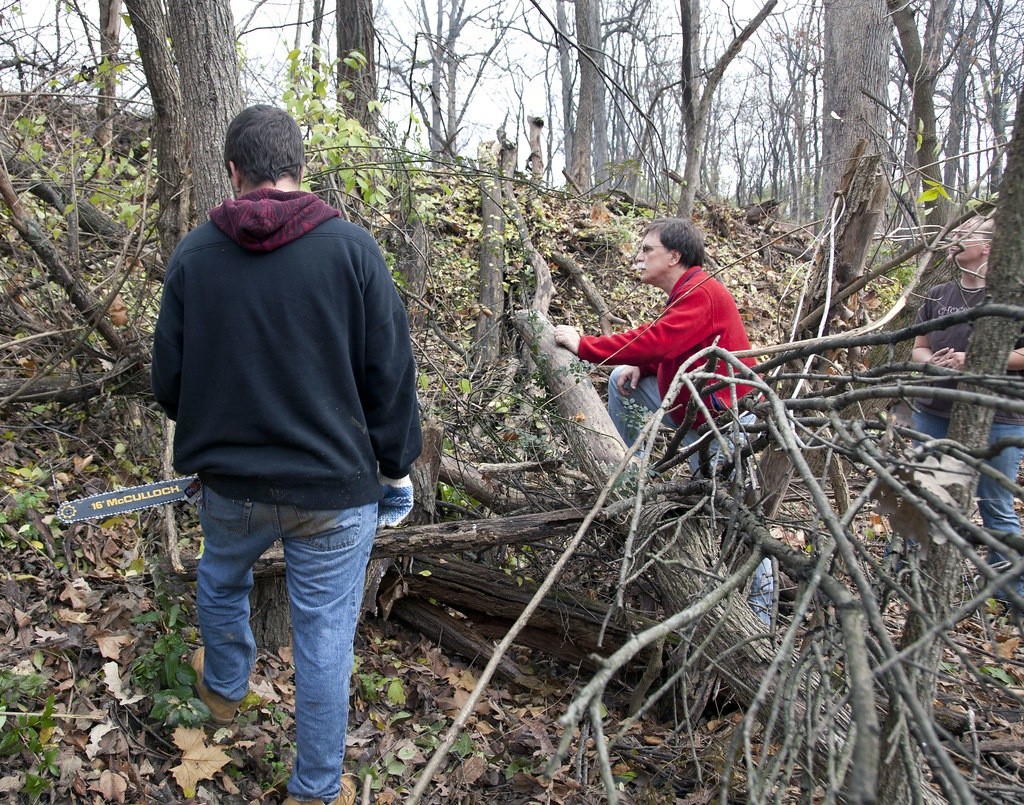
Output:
[641,244,665,252]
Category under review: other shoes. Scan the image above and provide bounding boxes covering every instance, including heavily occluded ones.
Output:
[191,645,242,724]
[282,776,356,805]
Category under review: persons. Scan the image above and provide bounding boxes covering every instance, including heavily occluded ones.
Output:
[152,105,423,805]
[882,216,1024,614]
[552,218,780,642]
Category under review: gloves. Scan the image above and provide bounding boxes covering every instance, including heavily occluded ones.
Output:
[377,470,414,528]
